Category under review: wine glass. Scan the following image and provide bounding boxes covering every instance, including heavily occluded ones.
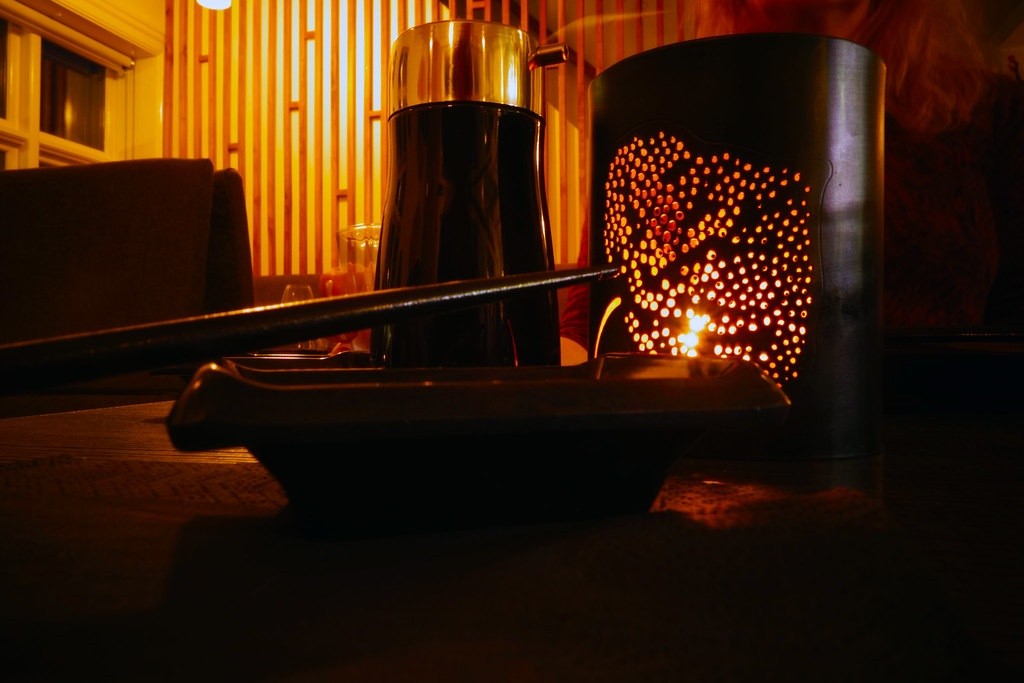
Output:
[281,285,314,349]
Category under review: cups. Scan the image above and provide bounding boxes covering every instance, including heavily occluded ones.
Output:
[337,226,381,290]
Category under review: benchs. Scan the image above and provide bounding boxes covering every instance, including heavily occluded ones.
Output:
[0,157,215,418]
[214,166,329,356]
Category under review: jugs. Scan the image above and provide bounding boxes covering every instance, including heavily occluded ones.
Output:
[369,19,567,371]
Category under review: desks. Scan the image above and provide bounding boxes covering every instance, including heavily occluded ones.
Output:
[2,394,698,681]
